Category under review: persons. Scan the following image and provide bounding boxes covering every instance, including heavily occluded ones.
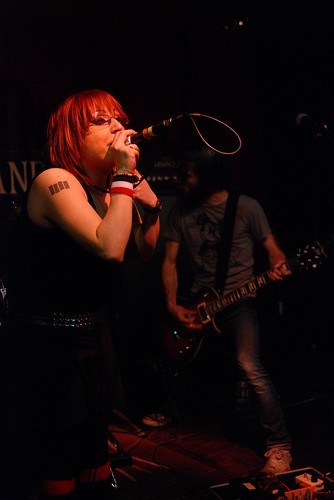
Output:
[23,90,164,500]
[161,162,291,471]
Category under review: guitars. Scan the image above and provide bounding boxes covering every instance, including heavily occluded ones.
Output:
[160,240,328,367]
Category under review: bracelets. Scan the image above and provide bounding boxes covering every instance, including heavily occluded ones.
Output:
[110,174,133,197]
[144,198,163,213]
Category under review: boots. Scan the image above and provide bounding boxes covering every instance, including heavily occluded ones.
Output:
[45,468,119,500]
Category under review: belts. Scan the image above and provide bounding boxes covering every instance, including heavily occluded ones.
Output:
[8,306,106,329]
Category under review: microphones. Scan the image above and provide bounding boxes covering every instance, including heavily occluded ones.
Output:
[123,116,184,144]
[297,113,334,137]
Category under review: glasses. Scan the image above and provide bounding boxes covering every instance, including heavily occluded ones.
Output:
[86,109,129,128]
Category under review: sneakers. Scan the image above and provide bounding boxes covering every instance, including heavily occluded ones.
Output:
[142,410,171,426]
[251,448,292,484]
